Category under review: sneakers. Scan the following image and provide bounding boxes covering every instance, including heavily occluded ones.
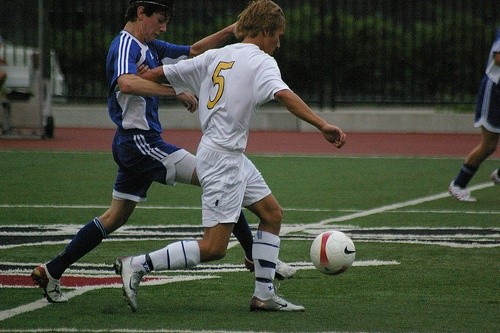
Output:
[249,293,305,312]
[113,256,142,313]
[31,263,68,302]
[491,170,500,184]
[245,258,296,280]
[448,181,476,202]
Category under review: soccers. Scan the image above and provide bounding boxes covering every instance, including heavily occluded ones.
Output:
[309,230,357,276]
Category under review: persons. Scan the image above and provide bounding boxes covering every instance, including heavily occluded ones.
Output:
[447,38,500,201]
[113,1,349,314]
[27,1,299,305]
